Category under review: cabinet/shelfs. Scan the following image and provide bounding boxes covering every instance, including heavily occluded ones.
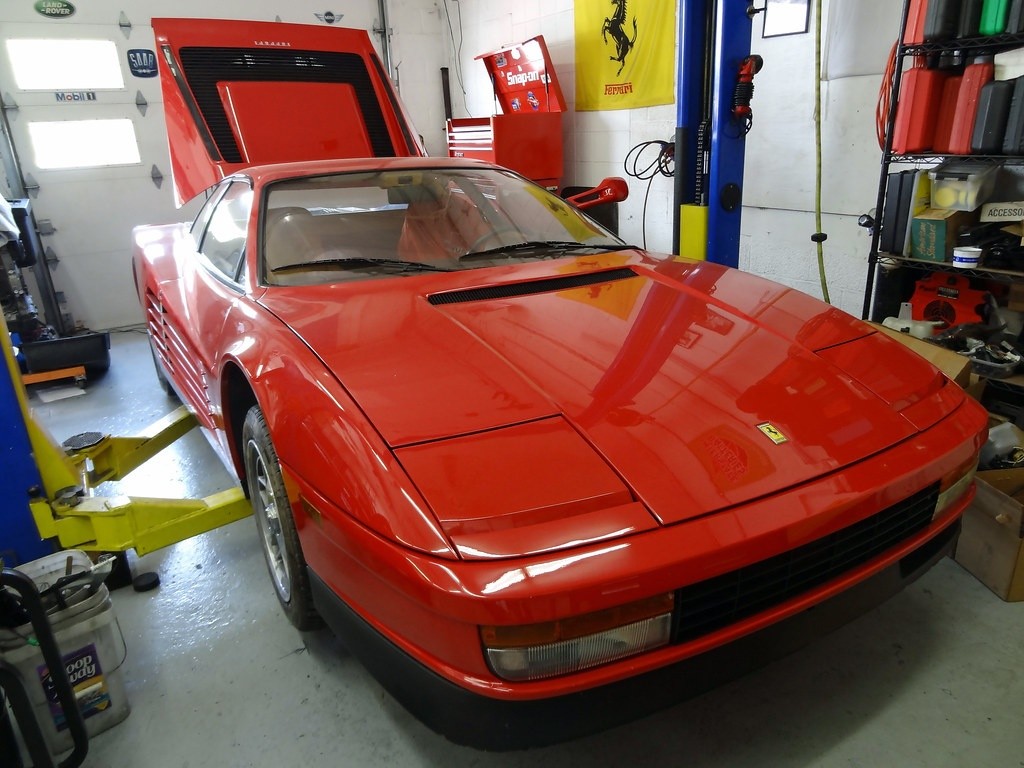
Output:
[858,0,1024,430]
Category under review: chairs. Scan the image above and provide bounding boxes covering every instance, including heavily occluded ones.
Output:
[398,192,490,262]
[261,207,327,271]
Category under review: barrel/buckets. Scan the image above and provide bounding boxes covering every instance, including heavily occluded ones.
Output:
[0,549,132,756]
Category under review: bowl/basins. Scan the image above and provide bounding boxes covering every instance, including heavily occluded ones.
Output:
[951,246,983,268]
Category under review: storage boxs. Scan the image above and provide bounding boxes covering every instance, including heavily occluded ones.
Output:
[860,318,974,391]
[928,157,1004,213]
[954,466,1024,602]
[910,207,978,262]
[880,166,918,253]
[889,0,1024,154]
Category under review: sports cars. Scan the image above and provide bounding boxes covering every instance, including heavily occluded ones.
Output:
[131,17,991,750]
